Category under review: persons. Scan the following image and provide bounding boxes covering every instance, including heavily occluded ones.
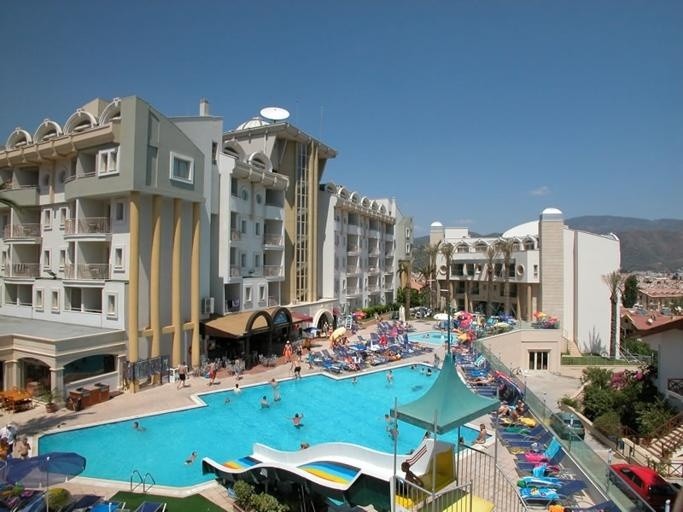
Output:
[494,400,524,423]
[469,376,494,385]
[261,396,270,408]
[133,422,145,432]
[185,451,197,464]
[390,423,399,439]
[288,413,302,427]
[274,392,281,402]
[177,320,439,394]
[18,438,31,460]
[384,414,392,427]
[458,437,464,447]
[424,431,431,441]
[0,423,16,458]
[301,443,309,449]
[473,424,486,445]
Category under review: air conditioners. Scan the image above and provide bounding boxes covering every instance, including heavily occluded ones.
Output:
[201,297,214,314]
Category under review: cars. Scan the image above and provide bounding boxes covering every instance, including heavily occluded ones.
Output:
[608,462,680,508]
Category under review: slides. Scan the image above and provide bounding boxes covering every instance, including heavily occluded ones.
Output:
[201,440,456,511]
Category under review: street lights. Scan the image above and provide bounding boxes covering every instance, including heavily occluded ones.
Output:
[509,361,512,377]
[569,415,574,454]
[524,372,527,400]
[606,448,613,498]
[543,391,547,425]
[498,352,501,373]
[663,497,671,512]
[487,343,492,363]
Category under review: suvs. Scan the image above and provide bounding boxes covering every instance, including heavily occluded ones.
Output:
[550,411,585,441]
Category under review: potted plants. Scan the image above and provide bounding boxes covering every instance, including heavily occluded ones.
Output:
[36,386,60,412]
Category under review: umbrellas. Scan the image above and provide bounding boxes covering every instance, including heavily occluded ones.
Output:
[5,453,87,512]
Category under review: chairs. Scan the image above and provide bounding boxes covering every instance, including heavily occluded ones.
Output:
[191,353,277,377]
[447,343,522,400]
[0,385,33,414]
[305,320,433,373]
[488,400,621,511]
[0,479,168,512]
